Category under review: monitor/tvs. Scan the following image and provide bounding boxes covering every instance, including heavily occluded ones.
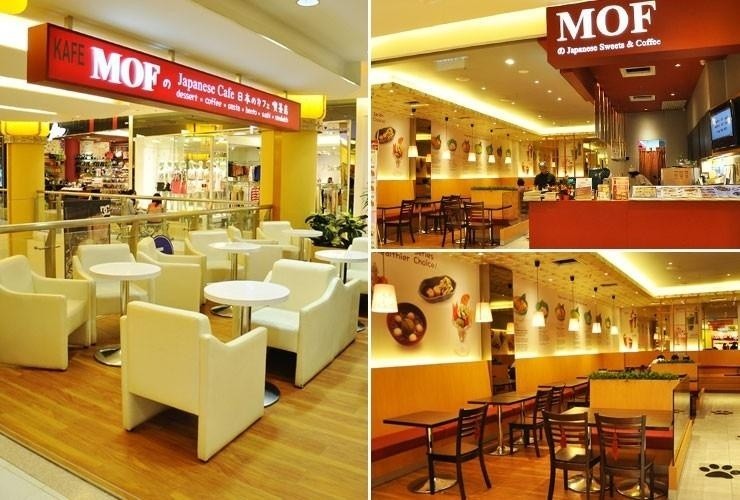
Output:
[589,169,603,189]
[568,178,576,184]
[709,97,740,153]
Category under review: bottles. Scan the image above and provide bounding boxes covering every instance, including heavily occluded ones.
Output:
[713,335,738,341]
[678,159,686,165]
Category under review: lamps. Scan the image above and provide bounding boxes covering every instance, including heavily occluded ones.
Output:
[371,253,622,337]
[407,107,514,166]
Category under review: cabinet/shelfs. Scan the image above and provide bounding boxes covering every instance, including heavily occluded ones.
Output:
[686,109,715,163]
[74,153,130,213]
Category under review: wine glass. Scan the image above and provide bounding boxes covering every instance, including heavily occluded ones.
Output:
[392,154,404,176]
[451,318,472,356]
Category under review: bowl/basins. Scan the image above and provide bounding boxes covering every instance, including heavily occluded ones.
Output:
[513,296,611,330]
[387,302,428,345]
[375,127,396,143]
[417,274,457,304]
[431,135,512,161]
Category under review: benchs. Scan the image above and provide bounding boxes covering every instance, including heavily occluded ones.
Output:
[697,362,740,393]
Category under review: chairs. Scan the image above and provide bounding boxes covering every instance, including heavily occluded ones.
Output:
[371,364,702,499]
[374,192,514,249]
[0,221,369,467]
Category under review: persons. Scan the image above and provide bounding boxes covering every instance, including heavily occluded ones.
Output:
[534,161,556,191]
[123,190,137,215]
[722,342,738,349]
[649,353,693,370]
[517,178,525,189]
[146,193,164,223]
[322,177,341,198]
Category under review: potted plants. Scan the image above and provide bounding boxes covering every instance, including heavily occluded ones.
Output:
[304,209,369,265]
